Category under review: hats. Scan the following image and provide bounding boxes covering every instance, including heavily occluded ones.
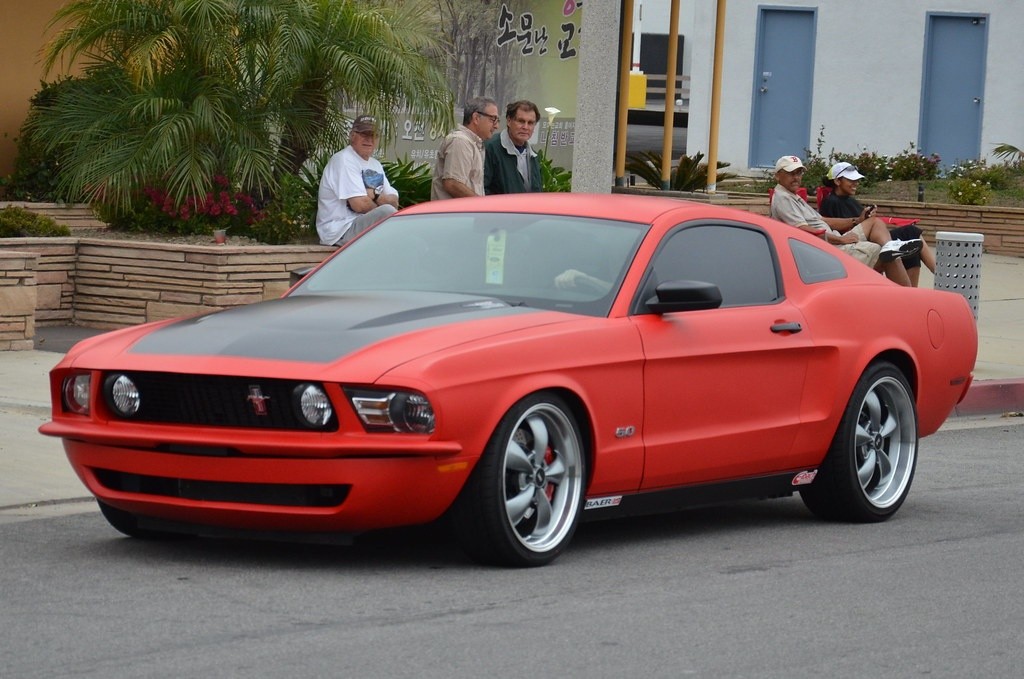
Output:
[827,162,865,180]
[352,115,381,137]
[775,156,807,173]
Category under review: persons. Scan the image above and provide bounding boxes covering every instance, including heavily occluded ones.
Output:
[484,100,542,194]
[555,269,612,291]
[771,156,923,287]
[820,162,934,287]
[430,96,499,201]
[316,116,399,247]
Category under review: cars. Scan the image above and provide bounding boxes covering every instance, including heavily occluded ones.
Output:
[38,190,979,570]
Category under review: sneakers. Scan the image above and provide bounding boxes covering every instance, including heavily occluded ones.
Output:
[879,238,923,262]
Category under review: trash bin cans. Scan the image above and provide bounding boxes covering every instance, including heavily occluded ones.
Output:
[933,232,985,327]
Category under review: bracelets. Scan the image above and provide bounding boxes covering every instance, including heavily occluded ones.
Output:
[852,218,857,226]
[373,190,381,201]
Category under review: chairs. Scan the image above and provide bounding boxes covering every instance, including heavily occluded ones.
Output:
[815,186,920,231]
[768,186,827,241]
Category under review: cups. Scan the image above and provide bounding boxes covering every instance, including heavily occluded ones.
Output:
[214,230,226,245]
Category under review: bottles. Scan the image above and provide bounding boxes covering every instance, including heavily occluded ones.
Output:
[918,183,924,202]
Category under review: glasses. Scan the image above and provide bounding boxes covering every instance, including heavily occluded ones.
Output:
[356,131,378,139]
[836,166,858,178]
[473,111,499,124]
